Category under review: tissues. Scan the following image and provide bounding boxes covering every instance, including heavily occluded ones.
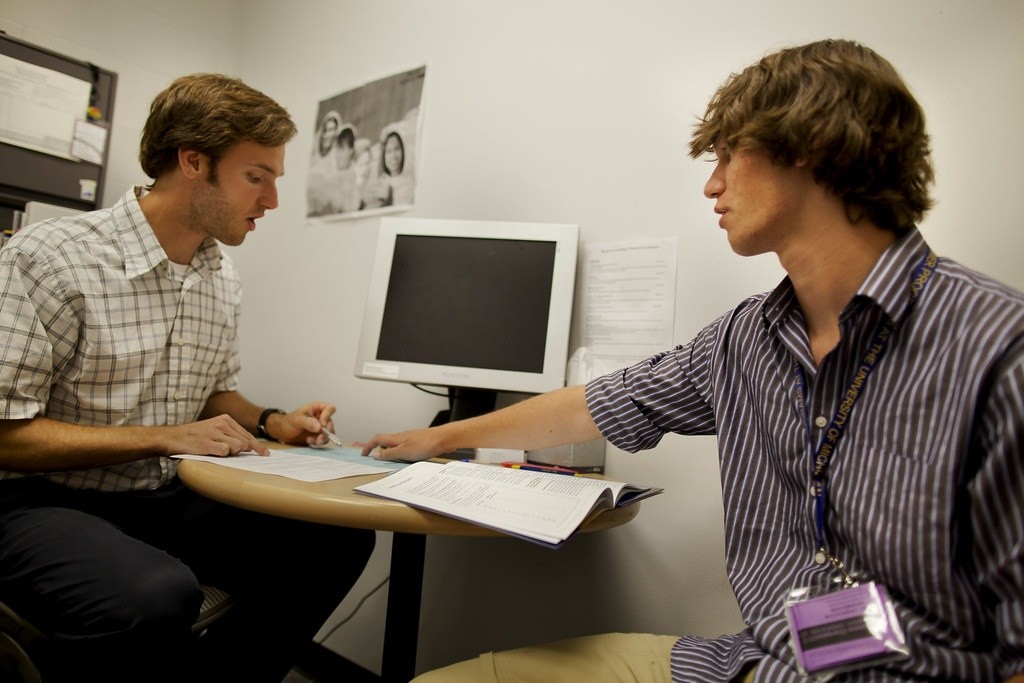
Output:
[525,345,607,474]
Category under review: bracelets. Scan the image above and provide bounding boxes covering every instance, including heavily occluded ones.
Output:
[257,408,288,443]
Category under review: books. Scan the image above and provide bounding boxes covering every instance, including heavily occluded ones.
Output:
[355,458,665,550]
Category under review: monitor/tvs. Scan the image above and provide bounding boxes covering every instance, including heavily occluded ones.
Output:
[351,215,580,460]
[0,32,120,212]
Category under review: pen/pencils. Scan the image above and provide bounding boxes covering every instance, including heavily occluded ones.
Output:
[501,463,580,476]
[321,427,342,446]
[461,458,490,465]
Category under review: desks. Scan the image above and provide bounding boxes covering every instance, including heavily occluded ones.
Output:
[175,440,641,683]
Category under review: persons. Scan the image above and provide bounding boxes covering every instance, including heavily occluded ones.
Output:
[376,120,415,208]
[363,40,1024,683]
[0,74,376,683]
[309,109,343,216]
[326,121,372,213]
